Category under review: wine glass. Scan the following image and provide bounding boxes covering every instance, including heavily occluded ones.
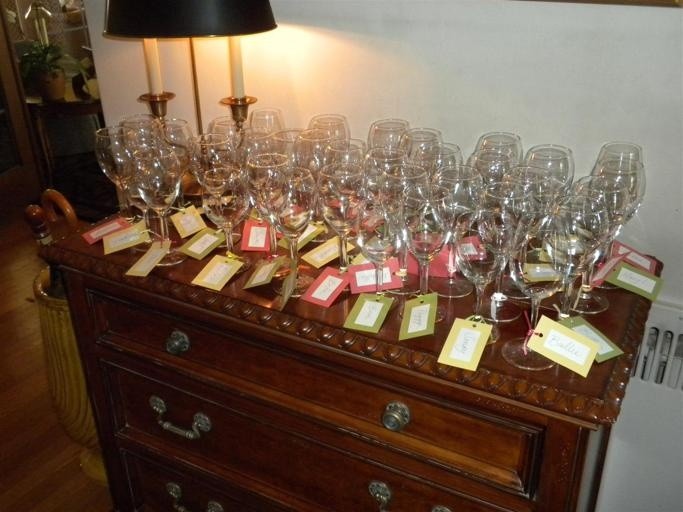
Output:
[94,109,646,370]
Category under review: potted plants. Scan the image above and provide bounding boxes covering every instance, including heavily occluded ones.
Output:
[14,41,90,102]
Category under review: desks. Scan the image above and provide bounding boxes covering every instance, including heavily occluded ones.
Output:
[25,71,120,221]
[37,205,665,511]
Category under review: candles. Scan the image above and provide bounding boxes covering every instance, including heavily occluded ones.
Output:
[140,38,162,97]
[227,36,246,101]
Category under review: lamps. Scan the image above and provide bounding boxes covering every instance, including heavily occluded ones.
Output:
[101,0,278,200]
